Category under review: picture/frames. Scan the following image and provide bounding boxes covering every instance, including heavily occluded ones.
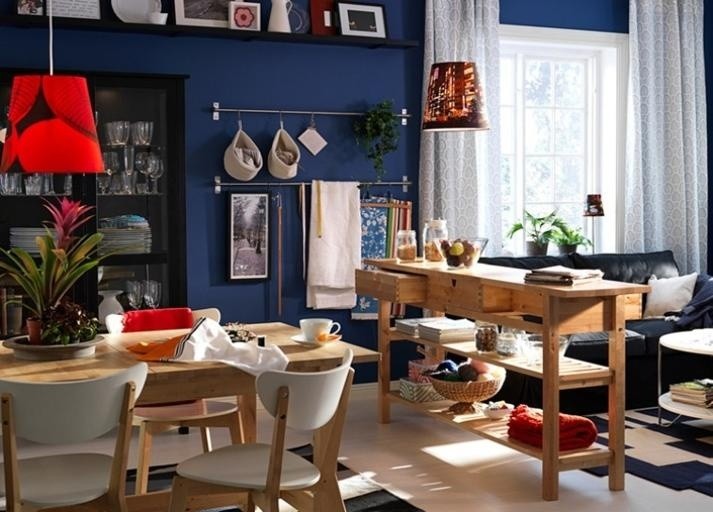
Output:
[222,189,271,283]
[14,0,46,20]
[311,1,333,35]
[229,2,261,32]
[334,0,390,39]
[175,1,229,28]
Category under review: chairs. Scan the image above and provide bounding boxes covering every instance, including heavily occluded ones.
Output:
[167,347,355,511]
[0,360,148,511]
[105,308,245,497]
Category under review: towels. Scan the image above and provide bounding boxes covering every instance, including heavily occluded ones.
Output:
[306,180,361,311]
[506,404,597,451]
[125,316,289,377]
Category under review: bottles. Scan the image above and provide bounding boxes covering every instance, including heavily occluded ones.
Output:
[395,229,417,263]
[423,220,449,262]
[267,0,293,33]
[470,319,528,356]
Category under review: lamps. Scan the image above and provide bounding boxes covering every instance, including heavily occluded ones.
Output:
[0,1,104,173]
[422,0,492,132]
[582,195,605,255]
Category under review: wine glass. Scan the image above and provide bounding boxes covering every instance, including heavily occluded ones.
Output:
[124,278,162,311]
[99,147,163,194]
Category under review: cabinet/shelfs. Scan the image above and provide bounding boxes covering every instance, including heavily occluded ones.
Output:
[0,71,186,338]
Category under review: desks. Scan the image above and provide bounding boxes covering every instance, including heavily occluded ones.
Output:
[444,251,713,414]
[355,256,652,501]
[658,328,713,427]
[0,321,383,512]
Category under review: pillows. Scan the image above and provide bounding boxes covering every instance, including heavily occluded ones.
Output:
[642,272,697,320]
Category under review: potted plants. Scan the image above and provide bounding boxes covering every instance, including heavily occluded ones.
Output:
[2,303,105,359]
[506,210,557,255]
[547,219,594,255]
[0,196,113,341]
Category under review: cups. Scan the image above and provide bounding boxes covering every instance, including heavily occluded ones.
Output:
[151,12,168,24]
[103,120,153,145]
[0,172,71,196]
[300,319,341,341]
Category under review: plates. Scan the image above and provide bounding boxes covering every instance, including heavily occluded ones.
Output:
[111,0,162,23]
[292,334,340,348]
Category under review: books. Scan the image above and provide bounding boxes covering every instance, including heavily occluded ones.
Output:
[394,315,476,344]
[523,264,603,286]
[668,377,713,409]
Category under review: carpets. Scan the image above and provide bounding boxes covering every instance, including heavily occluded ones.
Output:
[43,443,421,512]
[578,407,713,496]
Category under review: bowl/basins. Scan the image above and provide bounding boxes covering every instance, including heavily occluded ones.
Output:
[520,334,570,368]
[483,403,515,420]
[434,237,490,271]
